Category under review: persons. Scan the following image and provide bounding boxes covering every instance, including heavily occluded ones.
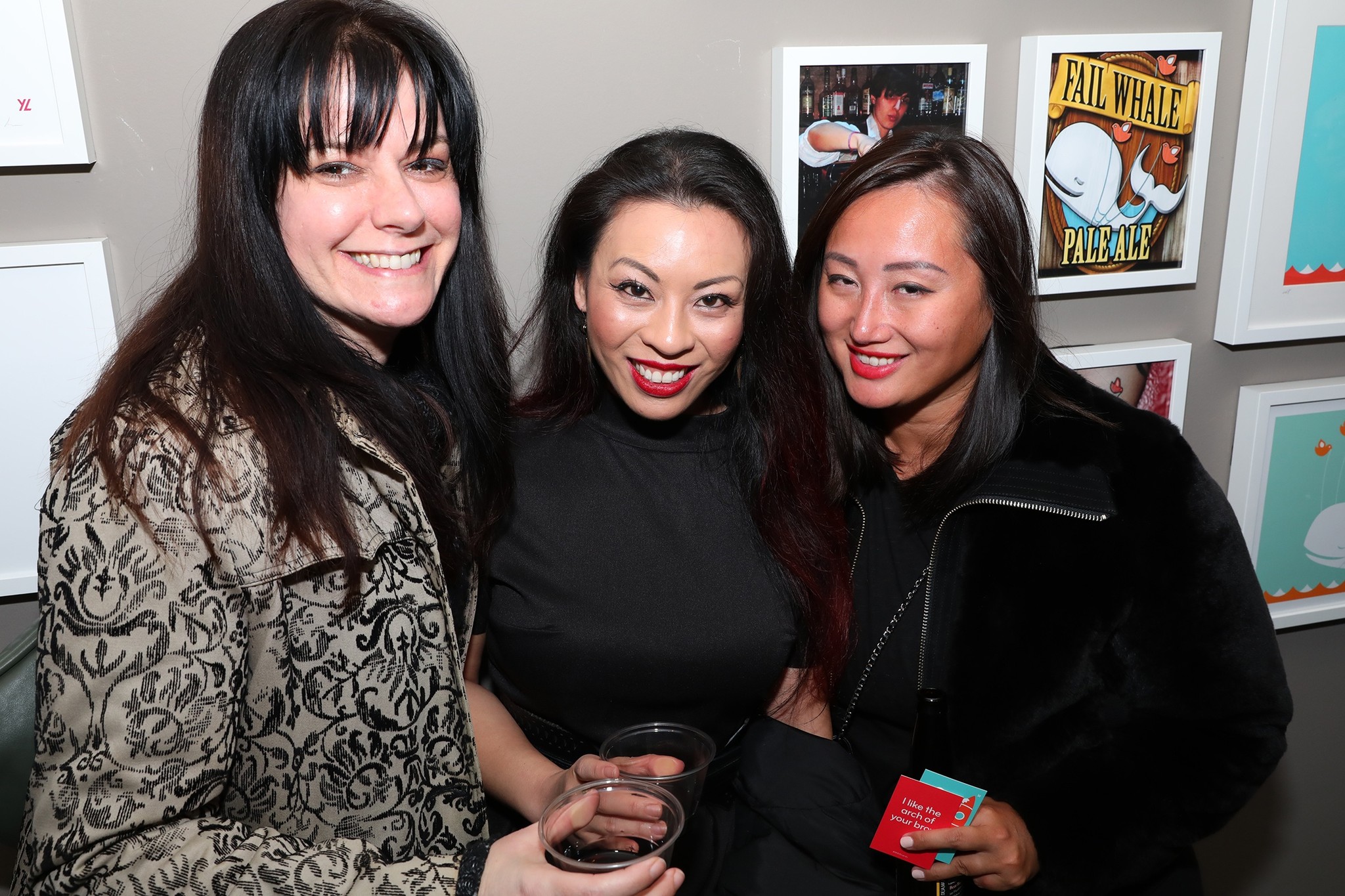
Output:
[799,63,916,190]
[799,128,1295,896]
[468,130,906,896]
[9,0,683,896]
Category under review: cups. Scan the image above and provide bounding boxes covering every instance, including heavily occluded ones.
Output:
[538,777,686,875]
[600,722,717,819]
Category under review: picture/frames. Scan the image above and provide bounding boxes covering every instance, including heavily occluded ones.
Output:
[1014,31,1223,294]
[1051,337,1191,433]
[0,0,100,170]
[1228,379,1345,629]
[1214,0,1345,351]
[772,43,988,273]
[0,240,121,597]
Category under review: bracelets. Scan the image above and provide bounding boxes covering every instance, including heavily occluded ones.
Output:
[848,131,862,151]
